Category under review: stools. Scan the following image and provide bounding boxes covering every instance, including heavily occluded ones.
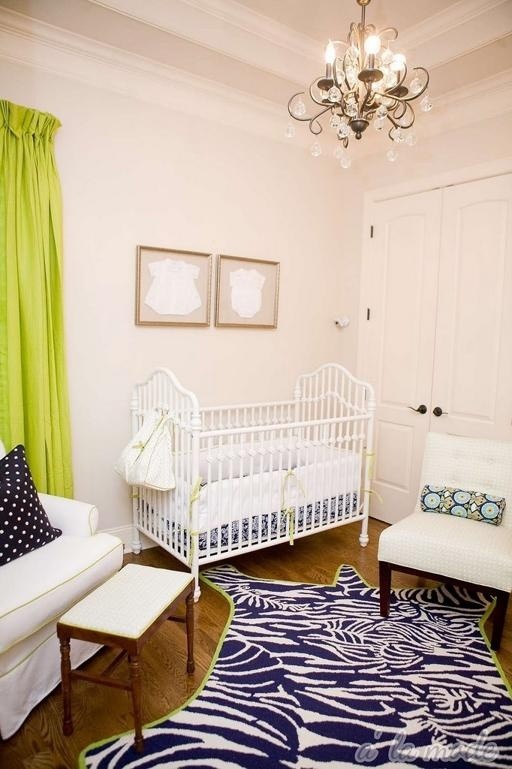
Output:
[57,562,194,750]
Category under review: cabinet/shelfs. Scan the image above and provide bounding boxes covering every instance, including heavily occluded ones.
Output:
[359,156,512,524]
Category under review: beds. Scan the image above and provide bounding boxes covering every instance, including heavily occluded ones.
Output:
[128,363,376,603]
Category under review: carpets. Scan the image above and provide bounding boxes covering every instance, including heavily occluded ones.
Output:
[77,564,512,768]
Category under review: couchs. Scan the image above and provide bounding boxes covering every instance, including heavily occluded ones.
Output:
[0,440,124,739]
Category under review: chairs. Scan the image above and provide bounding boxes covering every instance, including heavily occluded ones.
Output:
[377,432,512,651]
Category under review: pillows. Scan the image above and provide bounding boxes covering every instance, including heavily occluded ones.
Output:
[0,445,63,565]
[421,485,506,526]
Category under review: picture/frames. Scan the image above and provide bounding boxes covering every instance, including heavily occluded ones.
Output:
[136,244,213,328]
[214,254,280,329]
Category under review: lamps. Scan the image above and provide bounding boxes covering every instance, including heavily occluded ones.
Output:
[284,0,433,168]
